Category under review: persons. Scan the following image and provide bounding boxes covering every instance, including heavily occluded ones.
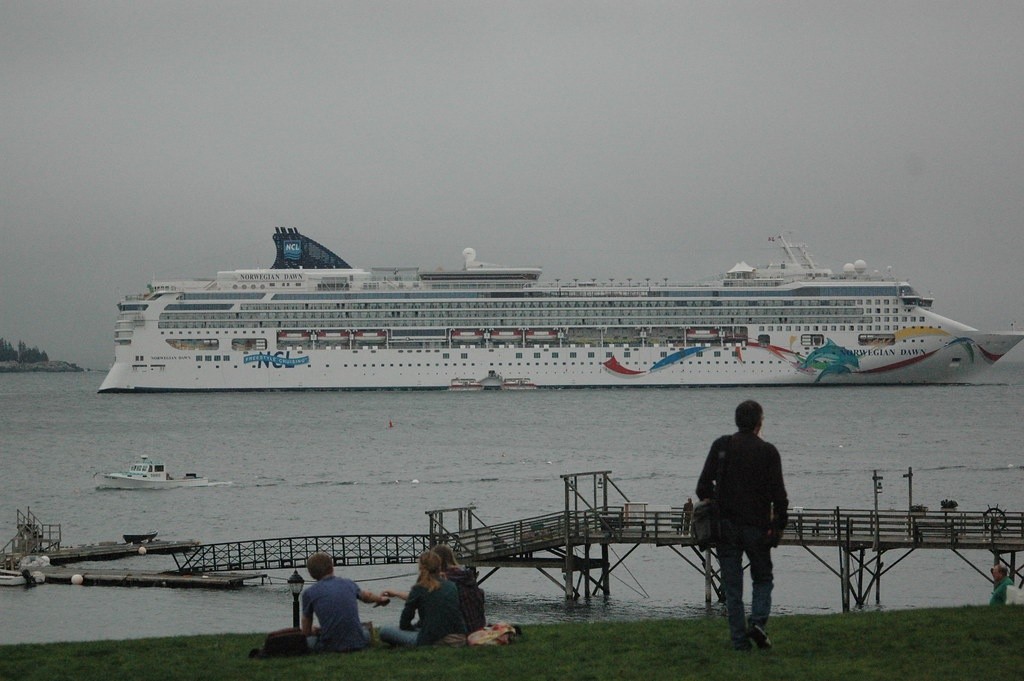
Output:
[379,544,486,646]
[683,498,693,535]
[695,400,789,650]
[300,552,391,653]
[990,565,1013,606]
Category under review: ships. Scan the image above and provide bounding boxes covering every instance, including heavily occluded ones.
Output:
[93,225,1024,390]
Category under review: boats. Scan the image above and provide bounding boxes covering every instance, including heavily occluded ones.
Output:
[451,328,485,344]
[354,332,386,345]
[524,330,558,342]
[687,328,718,341]
[92,454,208,489]
[490,331,522,343]
[317,331,348,345]
[277,331,311,343]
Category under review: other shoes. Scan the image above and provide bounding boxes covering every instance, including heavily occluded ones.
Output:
[748,622,772,650]
[735,641,754,651]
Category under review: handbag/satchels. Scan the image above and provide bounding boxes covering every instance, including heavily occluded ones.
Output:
[248,628,310,659]
[690,498,720,552]
[467,624,516,647]
